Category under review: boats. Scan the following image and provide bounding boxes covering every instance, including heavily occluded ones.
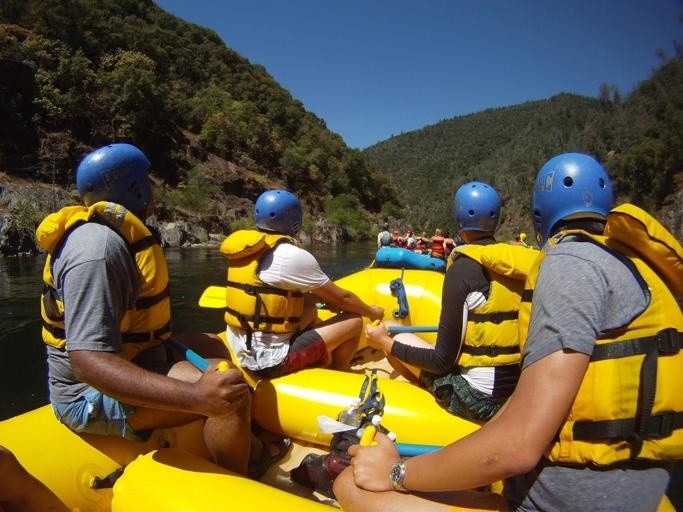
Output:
[374,246,446,272]
[0,261,674,511]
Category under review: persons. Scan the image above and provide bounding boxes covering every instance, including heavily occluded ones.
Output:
[377,223,465,261]
[297,152,683,512]
[219,188,384,380]
[36,143,292,476]
[365,182,541,420]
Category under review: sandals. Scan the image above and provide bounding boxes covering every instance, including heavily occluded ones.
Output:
[247,434,294,479]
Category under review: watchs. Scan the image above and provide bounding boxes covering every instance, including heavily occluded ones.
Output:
[390,460,408,494]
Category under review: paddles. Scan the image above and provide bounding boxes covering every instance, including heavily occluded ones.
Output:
[199,286,227,309]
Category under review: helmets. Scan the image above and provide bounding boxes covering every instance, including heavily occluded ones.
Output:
[254,189,303,238]
[453,181,502,236]
[531,150,615,245]
[76,142,153,214]
